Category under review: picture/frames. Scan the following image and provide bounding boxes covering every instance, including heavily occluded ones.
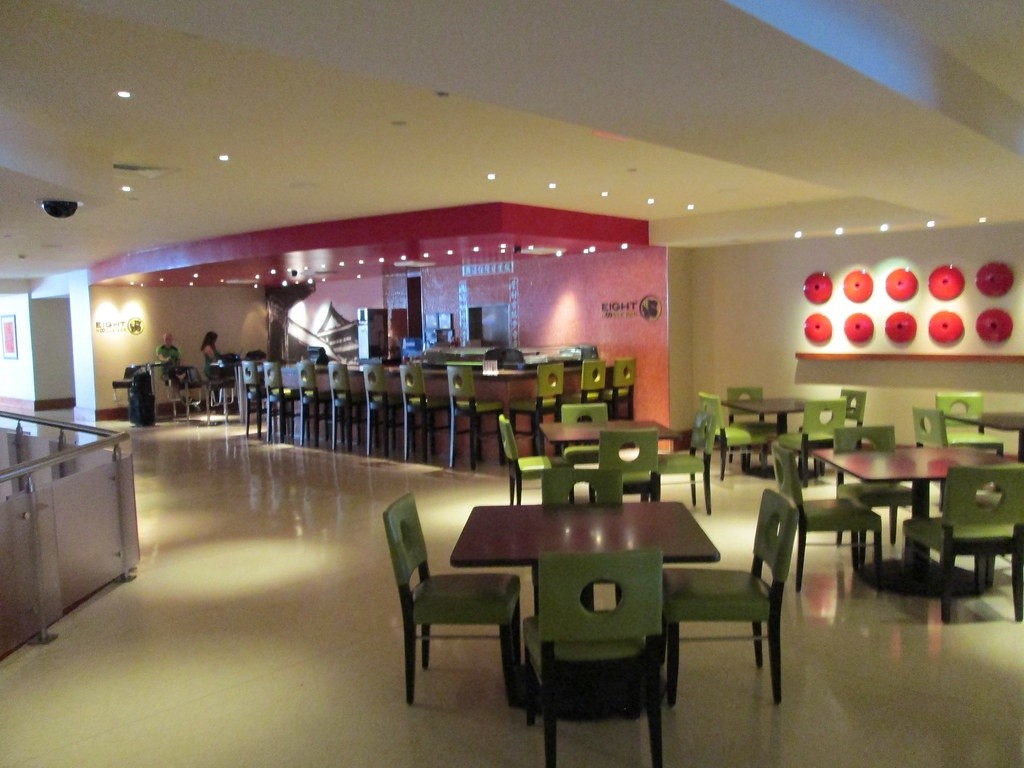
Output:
[1,315,18,361]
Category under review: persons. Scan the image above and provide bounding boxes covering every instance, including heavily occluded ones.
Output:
[200,331,220,397]
[156,331,181,398]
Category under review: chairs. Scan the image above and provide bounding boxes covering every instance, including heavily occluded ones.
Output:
[509,362,566,458]
[262,361,299,444]
[445,366,504,471]
[561,403,611,456]
[166,366,205,420]
[398,363,457,463]
[589,428,661,504]
[699,393,768,481]
[363,363,402,458]
[111,364,141,402]
[560,358,608,422]
[602,356,637,420]
[726,385,1024,626]
[327,361,365,452]
[245,349,267,361]
[500,415,574,507]
[205,354,239,425]
[241,360,266,439]
[295,361,331,449]
[383,487,799,768]
[652,392,723,514]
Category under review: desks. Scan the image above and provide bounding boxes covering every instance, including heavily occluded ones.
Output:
[811,447,1023,600]
[134,360,169,421]
[719,398,856,481]
[946,411,1024,462]
[449,502,722,728]
